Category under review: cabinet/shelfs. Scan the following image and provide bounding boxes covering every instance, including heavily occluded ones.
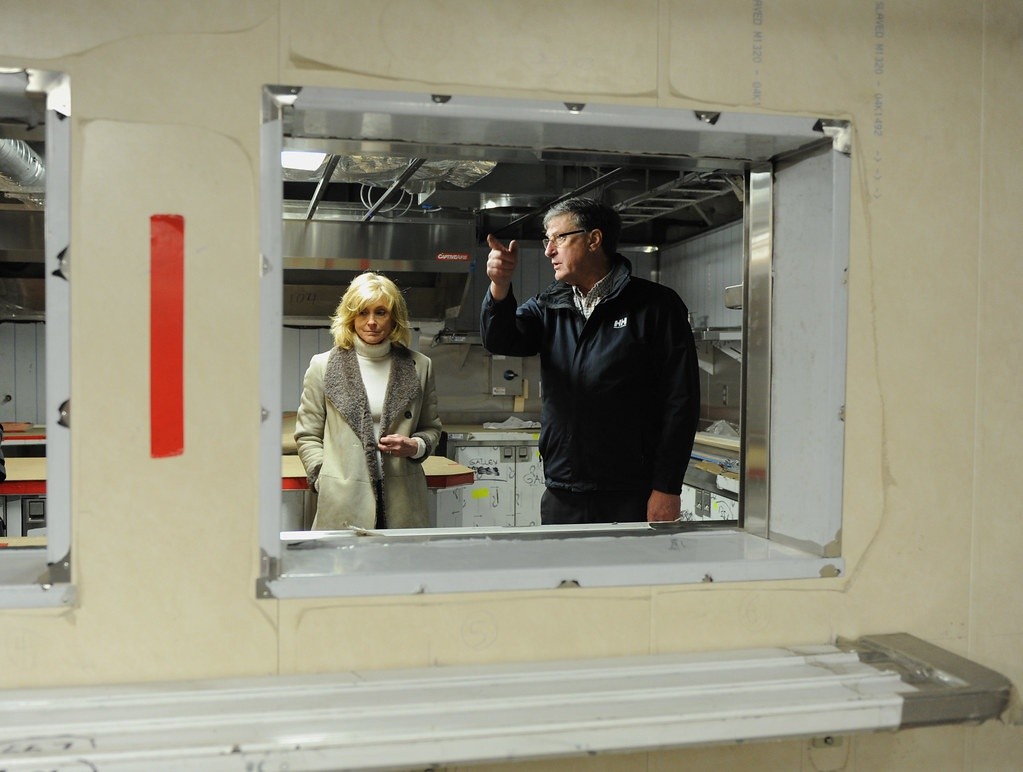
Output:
[431,448,738,529]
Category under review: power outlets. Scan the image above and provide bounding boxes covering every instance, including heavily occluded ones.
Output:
[722,384,729,406]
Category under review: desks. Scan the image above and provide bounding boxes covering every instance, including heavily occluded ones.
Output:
[0,454,476,536]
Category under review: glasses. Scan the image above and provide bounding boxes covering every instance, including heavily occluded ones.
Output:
[542,228,592,250]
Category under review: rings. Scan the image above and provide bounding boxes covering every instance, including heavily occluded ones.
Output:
[388,450,391,456]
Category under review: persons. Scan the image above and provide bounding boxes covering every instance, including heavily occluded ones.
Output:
[294,271,442,532]
[480,197,701,525]
